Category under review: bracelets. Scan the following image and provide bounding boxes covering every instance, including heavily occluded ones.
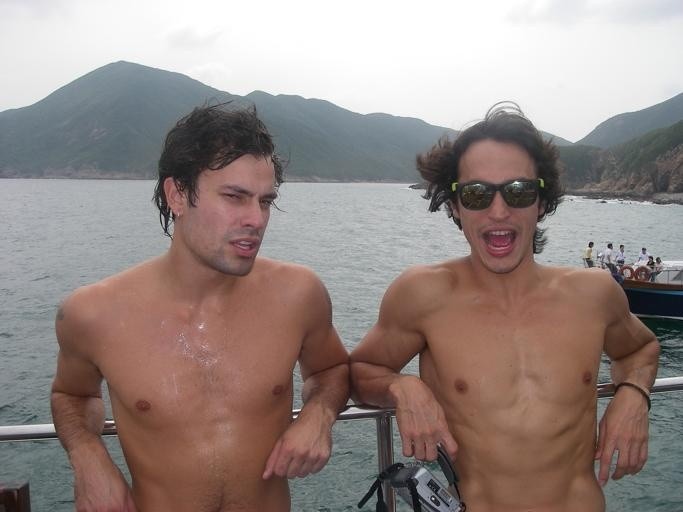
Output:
[614,380,652,411]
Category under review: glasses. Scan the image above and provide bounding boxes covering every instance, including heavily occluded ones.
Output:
[449,178,546,210]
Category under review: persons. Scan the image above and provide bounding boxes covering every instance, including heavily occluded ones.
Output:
[615,244,626,269]
[49,105,350,512]
[638,247,664,282]
[350,110,661,512]
[596,253,604,263]
[585,241,594,267]
[604,243,618,274]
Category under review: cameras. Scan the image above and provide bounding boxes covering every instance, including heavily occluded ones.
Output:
[390,465,465,512]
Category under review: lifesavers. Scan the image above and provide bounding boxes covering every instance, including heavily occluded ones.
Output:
[634,266,651,281]
[620,266,634,279]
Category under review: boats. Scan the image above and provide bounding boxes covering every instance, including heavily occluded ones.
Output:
[582,258,683,331]
